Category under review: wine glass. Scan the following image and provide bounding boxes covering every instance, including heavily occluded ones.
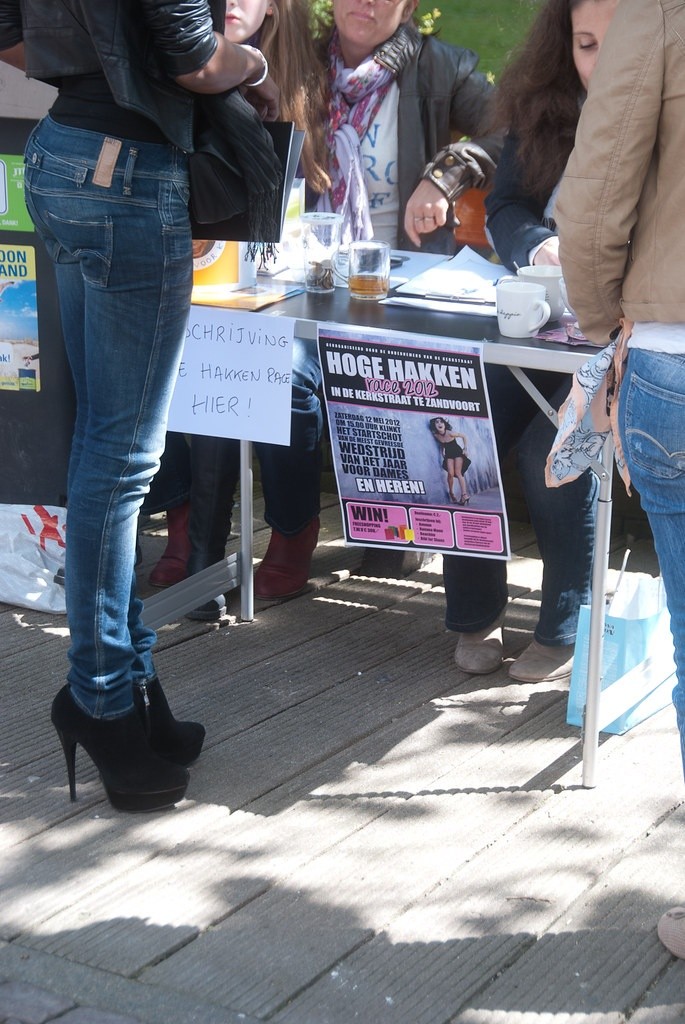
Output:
[557,277,587,341]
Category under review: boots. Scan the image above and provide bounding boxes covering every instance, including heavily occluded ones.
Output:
[185,434,240,620]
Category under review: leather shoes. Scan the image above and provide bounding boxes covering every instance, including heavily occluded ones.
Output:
[254,515,320,601]
[147,504,194,589]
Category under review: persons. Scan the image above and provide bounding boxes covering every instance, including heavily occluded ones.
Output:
[551,0,685,790]
[428,416,471,505]
[146,0,504,602]
[444,0,625,673]
[135,0,331,621]
[0,0,281,814]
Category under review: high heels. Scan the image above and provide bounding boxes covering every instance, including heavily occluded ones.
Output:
[454,494,470,506]
[132,678,206,767]
[51,684,190,811]
[451,495,458,504]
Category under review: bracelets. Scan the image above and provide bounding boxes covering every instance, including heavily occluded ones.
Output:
[239,43,268,87]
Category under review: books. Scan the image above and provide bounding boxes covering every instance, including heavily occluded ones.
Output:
[187,120,305,243]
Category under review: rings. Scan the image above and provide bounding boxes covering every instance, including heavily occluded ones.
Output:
[424,217,433,219]
[415,218,423,220]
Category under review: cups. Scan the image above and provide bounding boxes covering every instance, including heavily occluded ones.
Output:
[331,239,390,300]
[518,265,564,322]
[496,283,551,337]
[301,212,344,294]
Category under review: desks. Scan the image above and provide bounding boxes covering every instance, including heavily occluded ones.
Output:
[140,247,678,789]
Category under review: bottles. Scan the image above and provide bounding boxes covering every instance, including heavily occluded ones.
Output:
[333,243,349,288]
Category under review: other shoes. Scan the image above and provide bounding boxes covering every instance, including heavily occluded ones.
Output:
[657,907,685,961]
[454,598,508,674]
[508,639,575,684]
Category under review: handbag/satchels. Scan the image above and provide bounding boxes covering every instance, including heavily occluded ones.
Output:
[1,503,68,614]
[567,545,678,736]
[190,136,276,223]
